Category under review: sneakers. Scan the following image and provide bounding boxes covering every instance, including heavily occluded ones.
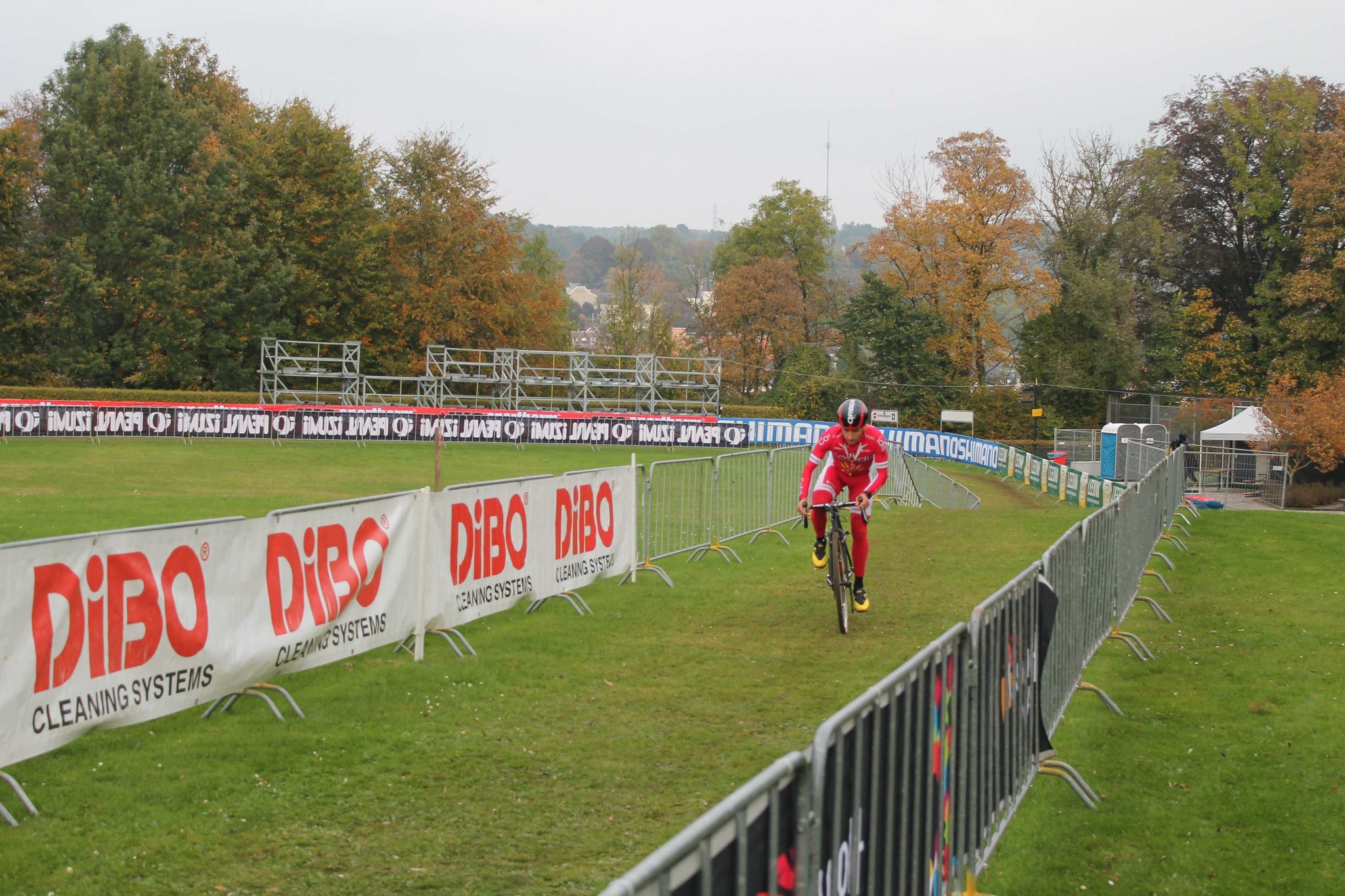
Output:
[812,536,828,568]
[853,587,869,612]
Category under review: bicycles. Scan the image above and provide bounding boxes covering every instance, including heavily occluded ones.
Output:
[802,496,869,635]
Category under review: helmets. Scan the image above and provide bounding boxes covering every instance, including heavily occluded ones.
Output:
[837,399,868,430]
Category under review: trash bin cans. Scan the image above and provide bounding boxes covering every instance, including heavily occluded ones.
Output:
[1047,451,1068,466]
[1185,496,1224,510]
[1100,422,1141,480]
[1134,422,1167,480]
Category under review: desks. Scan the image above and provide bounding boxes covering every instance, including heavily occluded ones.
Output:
[1070,461,1100,477]
[1201,467,1237,492]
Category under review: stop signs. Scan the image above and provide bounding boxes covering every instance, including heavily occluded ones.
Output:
[873,413,876,419]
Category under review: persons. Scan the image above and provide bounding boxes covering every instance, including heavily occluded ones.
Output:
[797,399,888,612]
[1181,441,1198,482]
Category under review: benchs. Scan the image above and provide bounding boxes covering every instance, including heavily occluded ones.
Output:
[1243,480,1261,492]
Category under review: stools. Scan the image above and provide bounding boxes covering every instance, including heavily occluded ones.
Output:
[1258,472,1269,491]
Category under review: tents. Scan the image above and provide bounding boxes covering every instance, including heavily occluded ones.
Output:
[1199,405,1298,492]
